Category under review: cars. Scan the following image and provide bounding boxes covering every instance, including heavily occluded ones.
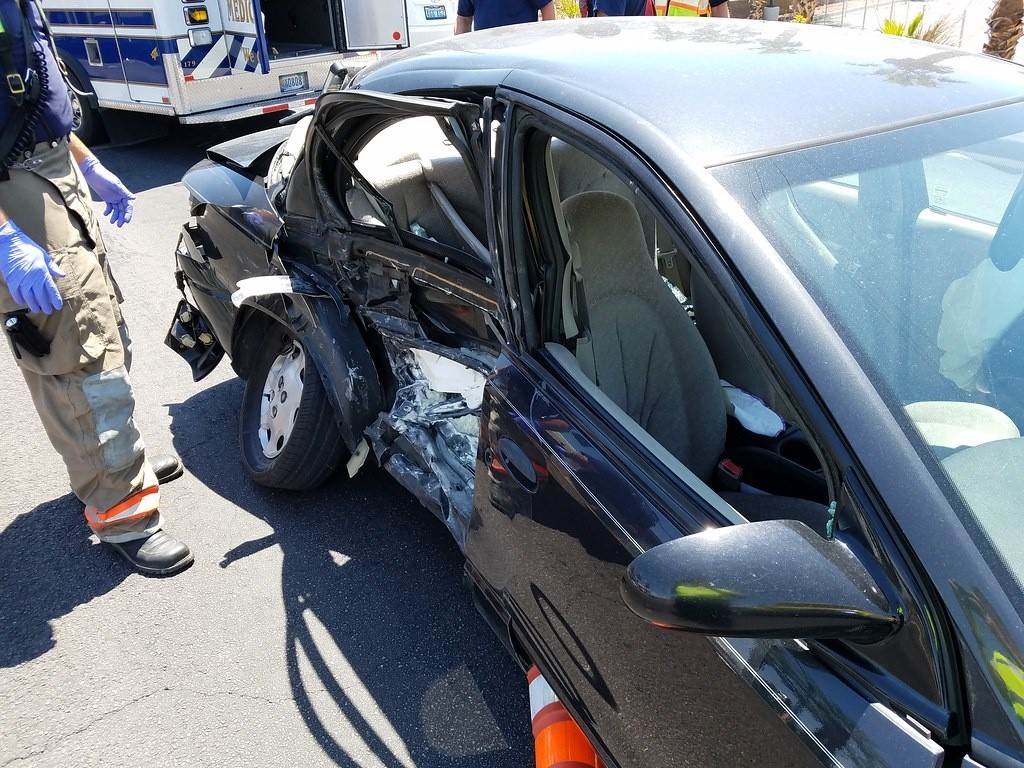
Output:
[161,14,1023,768]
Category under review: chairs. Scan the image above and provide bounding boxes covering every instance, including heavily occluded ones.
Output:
[559,191,838,541]
[689,189,1021,486]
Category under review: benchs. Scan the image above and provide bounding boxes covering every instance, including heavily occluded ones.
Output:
[346,138,674,279]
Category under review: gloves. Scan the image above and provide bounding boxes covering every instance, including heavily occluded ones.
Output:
[78,154,137,228]
[0,219,66,315]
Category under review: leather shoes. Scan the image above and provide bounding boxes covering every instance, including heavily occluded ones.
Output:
[69,453,184,508]
[99,527,195,575]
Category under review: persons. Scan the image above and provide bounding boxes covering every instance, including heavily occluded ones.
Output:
[485,352,588,519]
[670,573,747,630]
[0,0,194,578]
[454,0,730,35]
[947,579,1024,725]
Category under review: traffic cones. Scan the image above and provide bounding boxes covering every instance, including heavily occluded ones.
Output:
[525,663,607,768]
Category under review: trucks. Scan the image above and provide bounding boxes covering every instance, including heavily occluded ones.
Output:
[34,0,412,148]
